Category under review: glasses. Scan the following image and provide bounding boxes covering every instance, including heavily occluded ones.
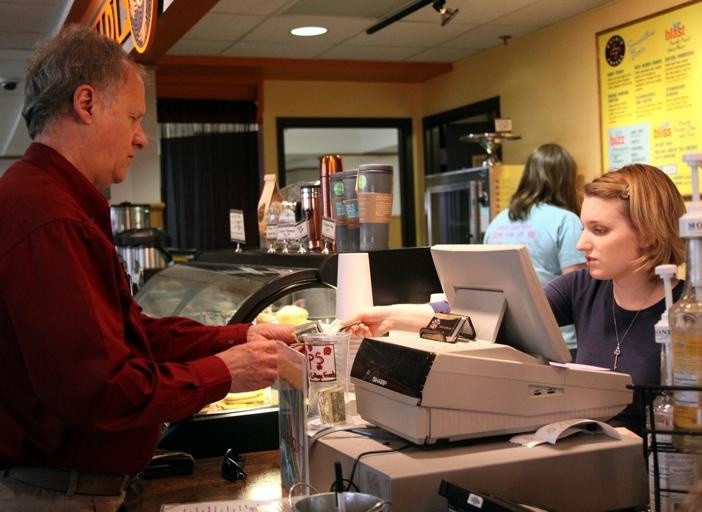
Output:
[8,464,140,496]
[220,447,246,480]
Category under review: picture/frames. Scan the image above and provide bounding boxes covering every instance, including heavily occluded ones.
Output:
[596,1,702,201]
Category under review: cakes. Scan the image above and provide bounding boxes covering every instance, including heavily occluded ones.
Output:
[276,304,310,328]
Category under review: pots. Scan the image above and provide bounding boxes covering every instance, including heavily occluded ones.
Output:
[288,482,394,512]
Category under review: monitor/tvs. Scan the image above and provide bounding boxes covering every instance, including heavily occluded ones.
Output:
[430,243,572,363]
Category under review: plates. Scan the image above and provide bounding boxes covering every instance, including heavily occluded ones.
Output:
[192,404,282,417]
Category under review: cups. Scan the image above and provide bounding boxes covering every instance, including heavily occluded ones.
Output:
[301,332,352,418]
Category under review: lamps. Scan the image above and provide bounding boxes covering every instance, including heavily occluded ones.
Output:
[432,2,459,26]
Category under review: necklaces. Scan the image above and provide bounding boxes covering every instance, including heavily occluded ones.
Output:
[612,281,657,372]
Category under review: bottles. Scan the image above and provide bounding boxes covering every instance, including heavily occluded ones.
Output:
[645,263,702,512]
[319,154,343,252]
[666,148,702,454]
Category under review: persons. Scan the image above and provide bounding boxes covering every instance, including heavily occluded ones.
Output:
[0,27,302,512]
[342,164,687,456]
[484,143,587,365]
